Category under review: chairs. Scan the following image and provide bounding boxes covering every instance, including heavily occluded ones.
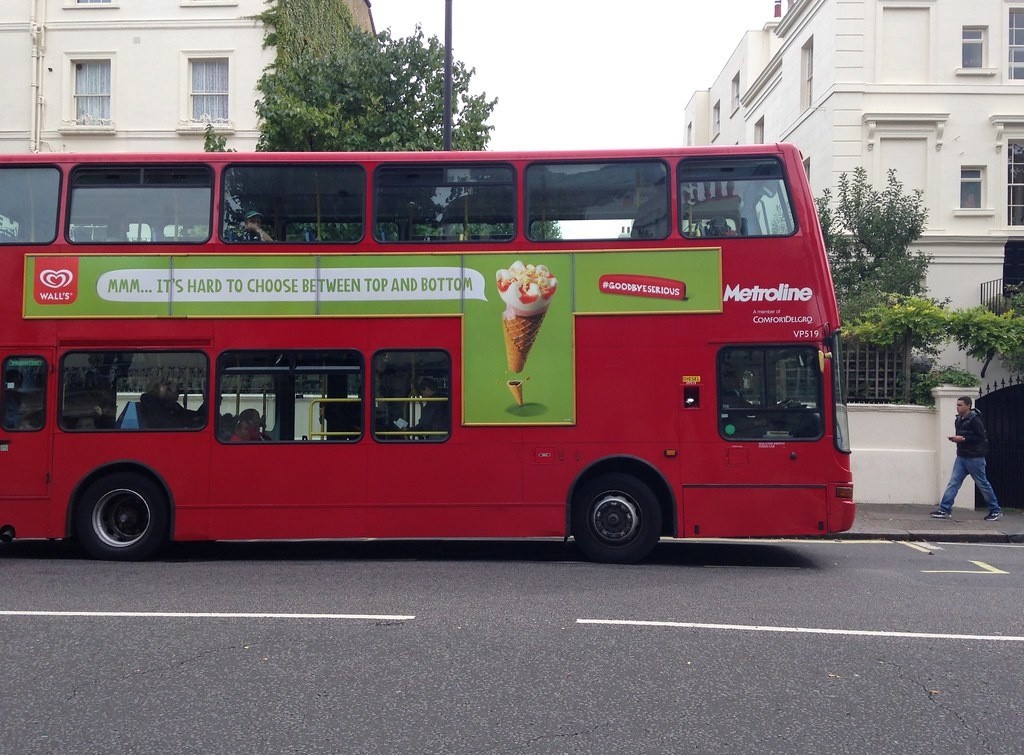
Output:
[140,394,172,428]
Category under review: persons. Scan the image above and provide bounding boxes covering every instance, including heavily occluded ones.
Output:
[401,378,449,439]
[104,213,131,242]
[5,369,33,428]
[721,363,789,409]
[154,379,267,442]
[240,210,279,242]
[929,396,1003,521]
[630,175,669,239]
[708,216,739,235]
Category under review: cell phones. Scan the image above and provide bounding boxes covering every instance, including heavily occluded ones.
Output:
[947,436,955,439]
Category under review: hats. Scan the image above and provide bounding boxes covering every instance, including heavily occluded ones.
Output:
[421,377,437,391]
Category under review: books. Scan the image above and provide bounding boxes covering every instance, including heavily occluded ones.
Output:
[392,418,408,430]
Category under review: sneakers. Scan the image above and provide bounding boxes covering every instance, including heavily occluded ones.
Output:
[929,509,951,519]
[984,511,1003,521]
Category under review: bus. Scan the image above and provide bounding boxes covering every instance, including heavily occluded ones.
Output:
[0,141,857,565]
